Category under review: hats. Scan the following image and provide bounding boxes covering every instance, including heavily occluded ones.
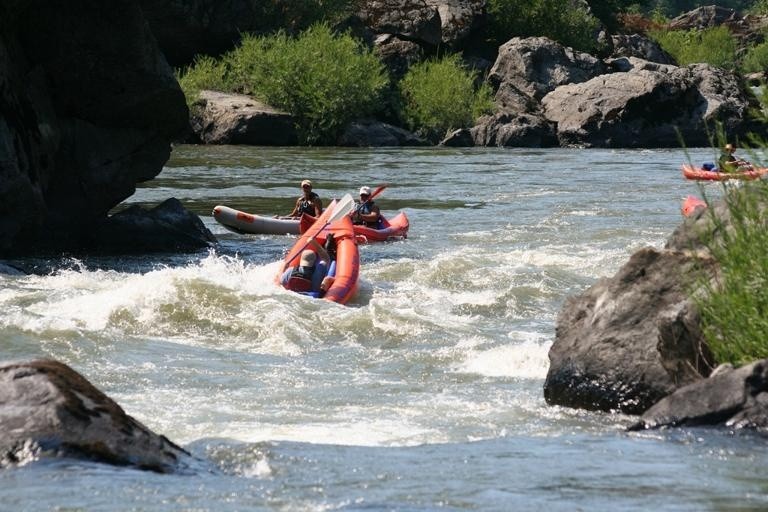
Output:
[359,186,370,196]
[300,250,315,266]
[302,180,312,187]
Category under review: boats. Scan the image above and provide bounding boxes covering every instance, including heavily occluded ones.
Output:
[681,195,707,218]
[275,197,360,304]
[212,205,325,234]
[300,211,408,243]
[682,163,768,182]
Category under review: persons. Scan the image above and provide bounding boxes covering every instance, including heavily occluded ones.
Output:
[350,186,380,230]
[719,144,751,172]
[274,238,330,298]
[289,179,323,220]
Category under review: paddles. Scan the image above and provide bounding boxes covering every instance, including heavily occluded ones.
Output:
[350,185,386,217]
[286,194,355,265]
